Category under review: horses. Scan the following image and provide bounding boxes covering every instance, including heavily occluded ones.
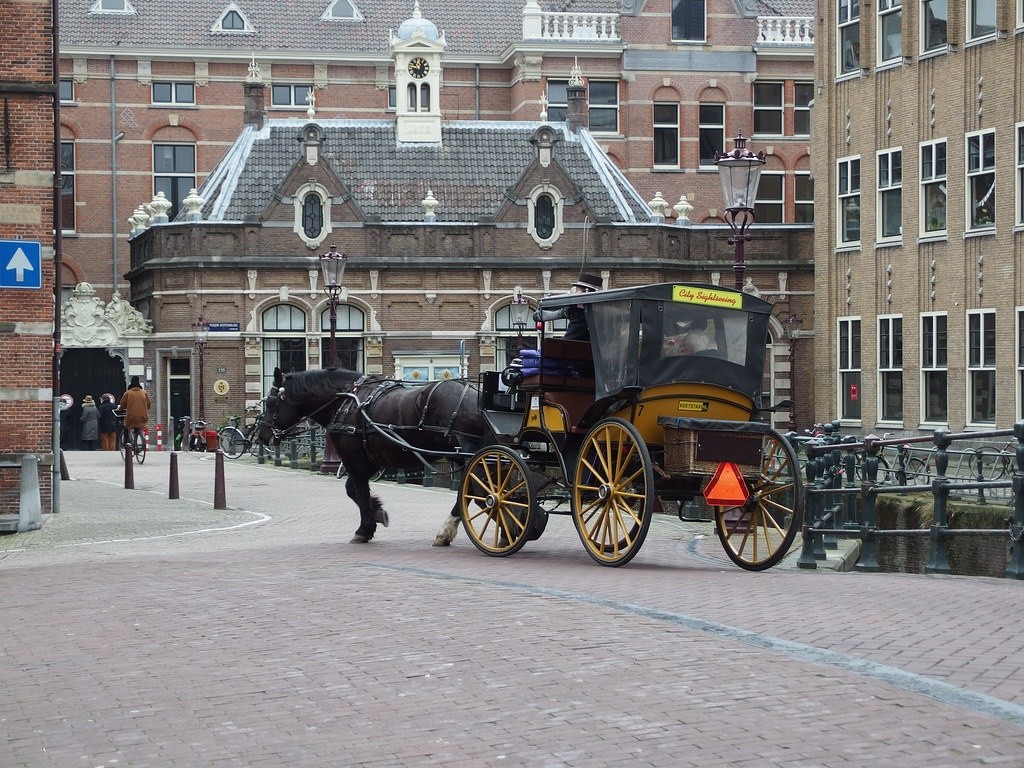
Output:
[257,367,516,547]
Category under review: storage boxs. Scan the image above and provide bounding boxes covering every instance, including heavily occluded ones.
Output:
[657,417,768,474]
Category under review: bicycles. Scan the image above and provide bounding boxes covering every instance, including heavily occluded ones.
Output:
[336,458,387,482]
[216,397,274,459]
[777,422,862,474]
[969,436,1017,482]
[856,432,931,486]
[112,408,148,464]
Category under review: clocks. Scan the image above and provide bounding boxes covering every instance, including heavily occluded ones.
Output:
[408,57,430,80]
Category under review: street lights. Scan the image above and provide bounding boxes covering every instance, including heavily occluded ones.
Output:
[714,128,766,291]
[318,238,348,476]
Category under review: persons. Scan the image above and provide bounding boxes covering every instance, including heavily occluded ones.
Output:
[97,395,118,451]
[80,395,101,451]
[115,375,151,449]
[60,398,76,451]
[552,273,603,342]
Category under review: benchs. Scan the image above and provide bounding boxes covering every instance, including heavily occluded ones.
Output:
[521,338,595,387]
[542,392,595,433]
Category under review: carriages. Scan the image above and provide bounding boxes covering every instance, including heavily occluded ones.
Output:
[257,281,804,571]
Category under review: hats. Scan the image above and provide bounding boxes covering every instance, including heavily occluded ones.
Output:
[82,395,94,403]
[572,274,603,291]
[99,394,110,400]
[128,376,143,391]
